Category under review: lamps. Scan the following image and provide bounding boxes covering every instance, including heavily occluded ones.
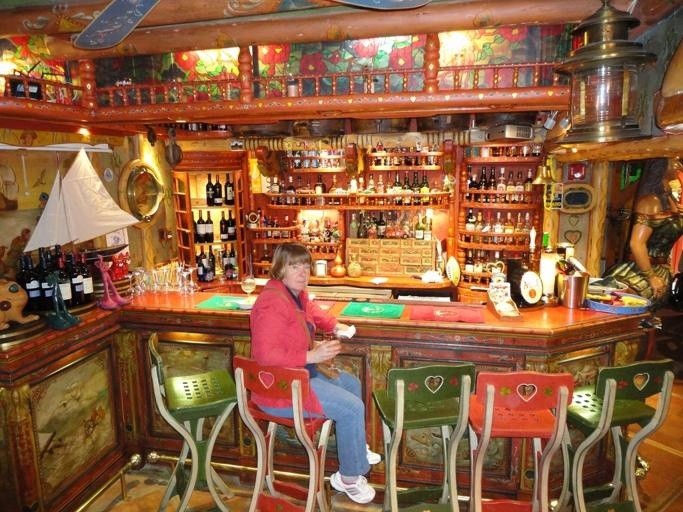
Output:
[558,0,659,144]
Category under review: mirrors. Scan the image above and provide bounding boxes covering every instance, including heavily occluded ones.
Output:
[117,159,166,229]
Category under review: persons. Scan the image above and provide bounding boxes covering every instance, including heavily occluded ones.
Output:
[607,157,683,305]
[249,242,382,503]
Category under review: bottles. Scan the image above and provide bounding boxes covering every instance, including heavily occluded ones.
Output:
[328,174,340,205]
[220,211,228,241]
[465,164,473,199]
[206,173,214,206]
[58,256,74,310]
[487,166,497,203]
[222,244,229,267]
[368,174,376,205]
[192,211,196,243]
[493,212,504,243]
[469,174,478,201]
[78,252,94,303]
[271,174,281,205]
[420,171,430,206]
[524,212,532,245]
[473,212,485,242]
[415,213,424,239]
[295,176,302,205]
[225,173,234,205]
[377,212,386,238]
[22,254,41,313]
[205,211,213,243]
[15,254,26,284]
[483,211,492,243]
[292,149,341,169]
[199,246,207,268]
[273,216,280,239]
[358,177,366,205]
[64,254,84,309]
[476,250,489,272]
[209,245,215,276]
[411,171,420,205]
[314,175,326,205]
[357,214,366,238]
[55,257,72,310]
[350,213,358,238]
[393,171,403,205]
[230,243,237,266]
[305,178,312,205]
[372,212,377,223]
[286,175,296,205]
[197,210,206,243]
[260,244,272,274]
[53,244,63,259]
[497,167,507,203]
[281,214,291,238]
[424,219,432,240]
[39,249,55,311]
[385,171,393,204]
[372,141,434,166]
[465,208,476,242]
[197,257,204,281]
[491,250,503,282]
[214,174,222,206]
[377,174,384,205]
[478,166,486,202]
[506,171,515,203]
[503,212,514,245]
[465,249,474,280]
[403,171,411,206]
[266,215,273,238]
[524,168,533,203]
[513,212,524,244]
[35,247,45,272]
[227,210,236,240]
[515,171,524,203]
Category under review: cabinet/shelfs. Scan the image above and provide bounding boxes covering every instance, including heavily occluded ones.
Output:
[165,150,246,288]
[459,141,547,287]
[245,142,457,279]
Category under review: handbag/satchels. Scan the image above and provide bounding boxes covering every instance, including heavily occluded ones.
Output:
[261,287,340,379]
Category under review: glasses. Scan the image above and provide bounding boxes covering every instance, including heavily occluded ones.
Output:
[285,265,315,274]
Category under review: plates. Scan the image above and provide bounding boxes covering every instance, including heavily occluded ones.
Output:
[520,270,543,304]
[586,293,651,314]
[588,276,628,293]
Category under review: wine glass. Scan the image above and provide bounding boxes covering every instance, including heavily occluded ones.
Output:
[242,275,256,307]
[126,264,198,300]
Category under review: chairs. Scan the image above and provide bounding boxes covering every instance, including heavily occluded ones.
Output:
[556,359,675,512]
[142,332,239,511]
[372,362,478,512]
[468,372,575,512]
[232,354,336,512]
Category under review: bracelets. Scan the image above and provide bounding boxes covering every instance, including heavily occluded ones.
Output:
[641,268,655,279]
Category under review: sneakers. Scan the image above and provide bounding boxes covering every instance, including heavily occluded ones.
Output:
[366,444,382,465]
[329,470,376,504]
